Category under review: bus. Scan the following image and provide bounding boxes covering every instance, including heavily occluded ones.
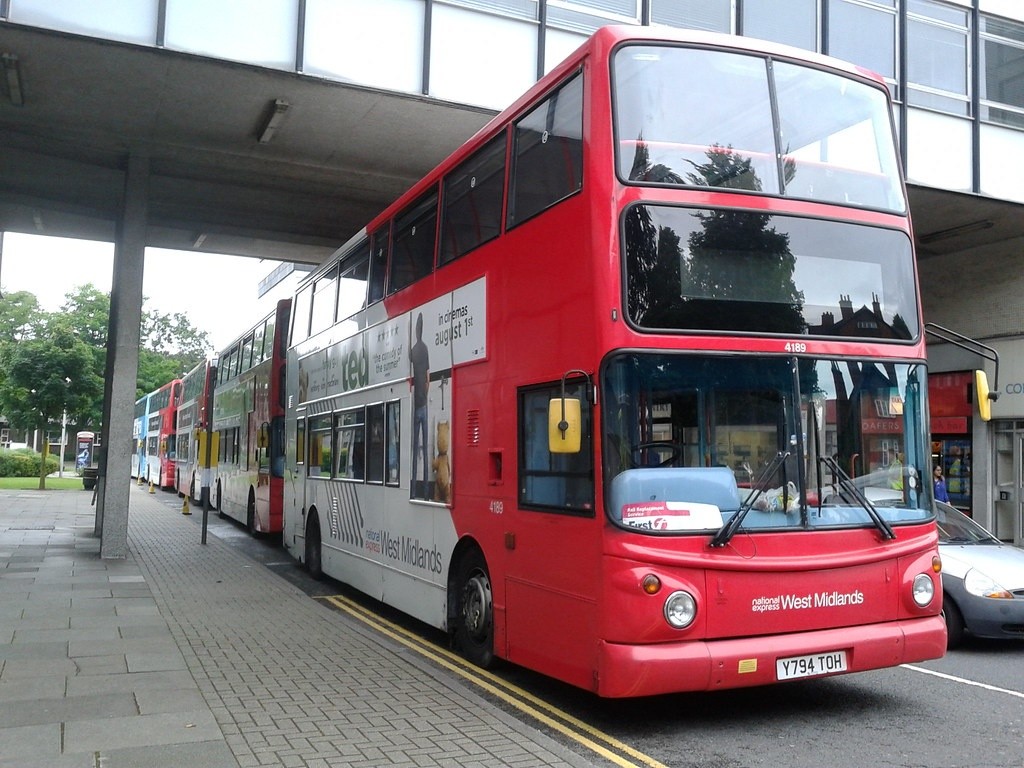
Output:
[173,356,219,506]
[208,297,294,542]
[281,21,1001,702]
[145,378,182,493]
[130,395,148,479]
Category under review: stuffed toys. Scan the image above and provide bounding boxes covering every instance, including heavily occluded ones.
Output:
[432,421,451,503]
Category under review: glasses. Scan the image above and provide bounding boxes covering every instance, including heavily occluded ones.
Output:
[936,469,942,471]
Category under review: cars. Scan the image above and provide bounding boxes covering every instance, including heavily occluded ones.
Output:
[931,498,1024,651]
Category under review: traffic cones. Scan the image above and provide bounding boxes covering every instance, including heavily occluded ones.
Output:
[181,495,192,515]
[137,474,144,486]
[149,479,155,494]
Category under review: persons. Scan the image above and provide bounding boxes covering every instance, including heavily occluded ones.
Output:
[880,448,903,501]
[932,463,950,522]
[409,313,430,502]
